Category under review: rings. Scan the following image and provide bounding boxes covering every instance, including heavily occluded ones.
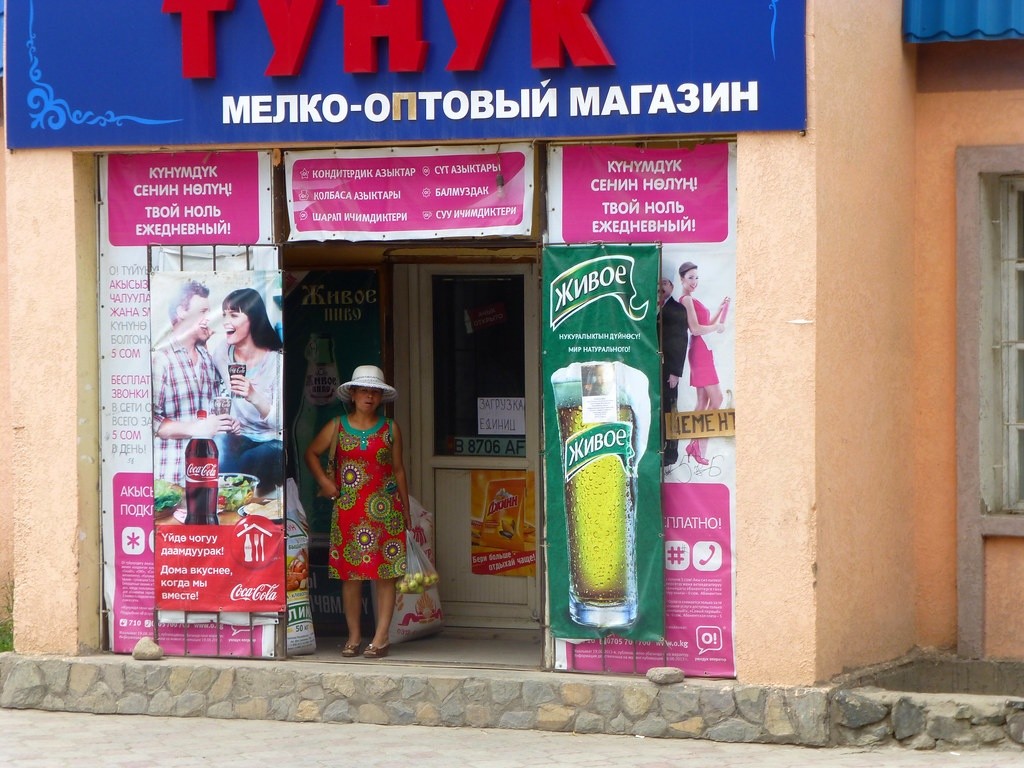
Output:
[331,497,334,500]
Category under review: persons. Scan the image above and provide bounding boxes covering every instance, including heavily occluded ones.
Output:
[678,261,730,464]
[306,365,411,657]
[662,278,688,467]
[152,279,283,486]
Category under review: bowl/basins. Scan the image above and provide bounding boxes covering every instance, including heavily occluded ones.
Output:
[217,472,261,511]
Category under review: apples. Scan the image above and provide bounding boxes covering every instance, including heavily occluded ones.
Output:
[395,572,439,594]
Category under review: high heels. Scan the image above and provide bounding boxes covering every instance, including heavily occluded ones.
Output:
[686,440,709,466]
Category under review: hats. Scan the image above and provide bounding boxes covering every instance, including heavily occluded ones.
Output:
[337,365,398,404]
[653,262,676,287]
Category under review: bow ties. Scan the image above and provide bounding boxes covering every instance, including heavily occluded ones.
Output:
[657,299,665,306]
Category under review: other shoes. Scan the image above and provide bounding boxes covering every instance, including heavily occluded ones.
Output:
[343,637,362,657]
[364,638,391,658]
[661,448,678,467]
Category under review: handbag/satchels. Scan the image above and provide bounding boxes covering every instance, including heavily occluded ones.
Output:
[395,528,440,594]
[312,416,340,534]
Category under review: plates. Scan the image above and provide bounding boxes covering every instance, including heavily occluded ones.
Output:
[238,505,283,525]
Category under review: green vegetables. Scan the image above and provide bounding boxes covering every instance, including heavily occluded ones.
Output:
[215,474,252,512]
[153,478,181,513]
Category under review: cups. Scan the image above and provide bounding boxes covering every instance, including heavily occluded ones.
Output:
[229,363,247,398]
[552,377,640,629]
[213,399,231,433]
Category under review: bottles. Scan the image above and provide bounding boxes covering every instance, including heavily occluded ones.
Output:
[184,410,219,526]
[293,332,348,537]
